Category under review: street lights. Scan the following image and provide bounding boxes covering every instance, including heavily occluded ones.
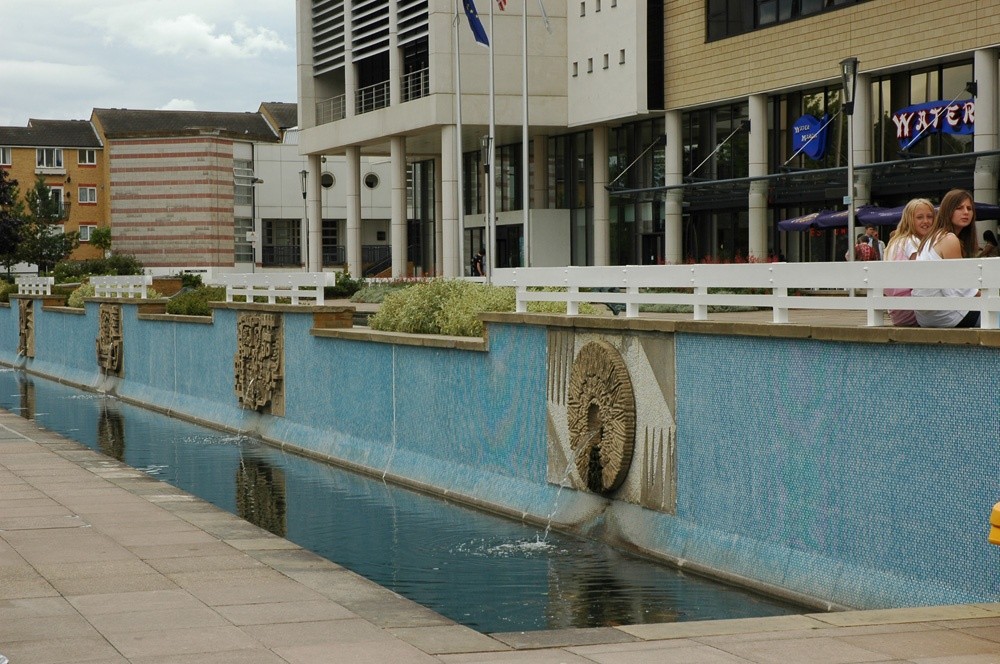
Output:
[297,169,309,272]
[479,135,494,285]
[839,57,861,297]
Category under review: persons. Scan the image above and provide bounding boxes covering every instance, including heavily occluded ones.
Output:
[882,198,936,327]
[470,248,486,277]
[911,189,982,328]
[845,221,885,291]
[977,228,999,258]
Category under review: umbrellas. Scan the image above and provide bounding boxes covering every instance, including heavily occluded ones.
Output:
[776,202,1000,262]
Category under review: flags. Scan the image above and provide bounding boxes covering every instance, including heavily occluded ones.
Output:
[496,0,507,11]
[463,0,490,48]
